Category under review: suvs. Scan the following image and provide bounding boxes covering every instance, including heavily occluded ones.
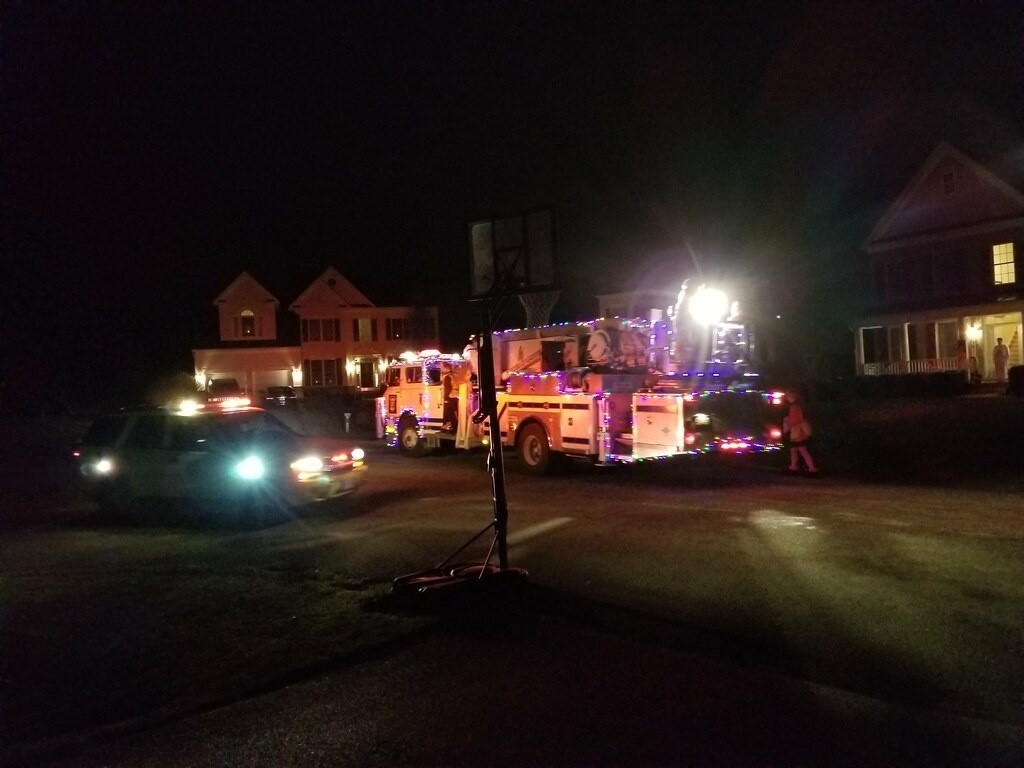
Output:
[75,403,367,529]
[202,378,244,404]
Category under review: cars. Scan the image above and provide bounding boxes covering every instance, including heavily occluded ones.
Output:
[262,386,298,410]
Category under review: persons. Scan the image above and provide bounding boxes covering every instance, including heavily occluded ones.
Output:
[781,391,818,474]
[993,337,1010,380]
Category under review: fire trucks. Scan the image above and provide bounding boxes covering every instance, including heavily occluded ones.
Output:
[371,319,790,478]
